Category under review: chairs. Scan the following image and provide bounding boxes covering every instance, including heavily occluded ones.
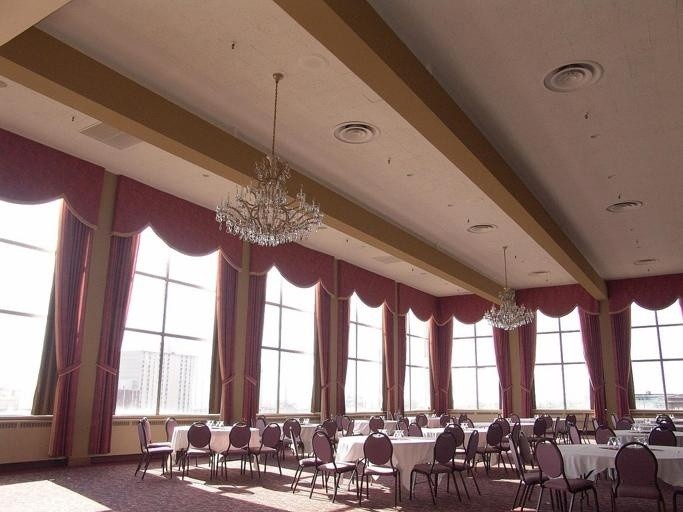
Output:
[442,424,466,494]
[609,414,617,428]
[507,414,519,423]
[434,430,480,501]
[394,410,402,421]
[527,418,545,452]
[308,432,359,504]
[592,418,599,431]
[594,426,615,444]
[615,418,633,430]
[568,424,581,444]
[619,415,633,423]
[141,417,172,472]
[399,415,408,427]
[497,422,521,471]
[242,423,281,478]
[394,421,408,437]
[671,486,682,512]
[134,417,173,481]
[471,423,506,476]
[368,415,384,434]
[255,416,283,461]
[386,410,393,420]
[414,413,427,428]
[450,416,457,427]
[439,414,450,428]
[282,419,304,462]
[408,423,422,437]
[312,419,336,457]
[433,408,446,417]
[655,414,671,424]
[648,427,676,446]
[165,416,197,471]
[341,415,362,436]
[409,432,461,506]
[493,417,514,472]
[358,432,400,507]
[658,420,676,431]
[539,414,556,443]
[578,412,589,444]
[215,422,253,482]
[533,438,598,512]
[557,414,576,444]
[497,413,502,421]
[290,425,329,495]
[505,434,554,512]
[458,414,473,428]
[334,416,349,431]
[518,431,560,509]
[181,423,212,481]
[553,416,559,440]
[608,442,664,512]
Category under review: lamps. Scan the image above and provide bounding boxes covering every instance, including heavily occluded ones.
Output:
[481,246,534,331]
[213,73,326,247]
